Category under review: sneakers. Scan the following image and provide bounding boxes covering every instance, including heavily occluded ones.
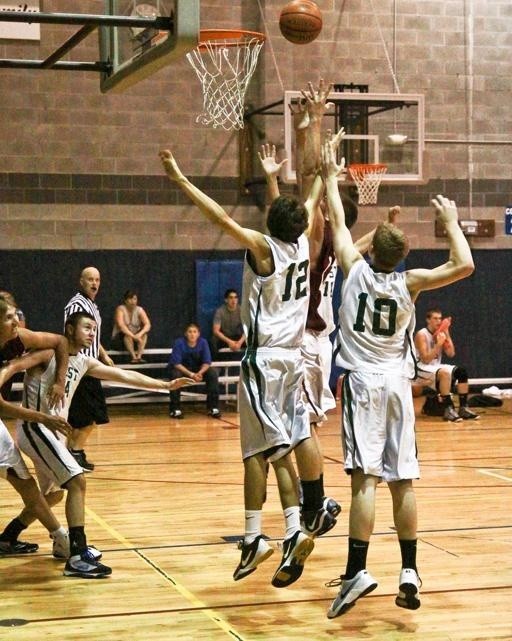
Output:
[0,532,111,579]
[458,407,480,420]
[206,407,221,418]
[70,448,94,471]
[234,495,421,618]
[169,410,183,419]
[444,407,464,422]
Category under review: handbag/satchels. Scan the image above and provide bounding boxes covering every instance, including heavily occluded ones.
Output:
[421,394,445,416]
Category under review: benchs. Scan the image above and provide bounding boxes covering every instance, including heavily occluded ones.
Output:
[429,374,512,386]
[3,347,240,405]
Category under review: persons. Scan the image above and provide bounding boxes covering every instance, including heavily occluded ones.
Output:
[314,141,475,617]
[1,292,102,561]
[111,289,152,364]
[210,288,247,362]
[259,79,359,537]
[415,309,479,423]
[1,311,195,576]
[166,324,222,419]
[158,126,346,589]
[63,267,114,473]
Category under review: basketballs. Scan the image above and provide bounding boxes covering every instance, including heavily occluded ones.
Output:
[279,2,323,45]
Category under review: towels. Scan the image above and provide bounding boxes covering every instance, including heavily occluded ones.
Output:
[482,384,512,399]
